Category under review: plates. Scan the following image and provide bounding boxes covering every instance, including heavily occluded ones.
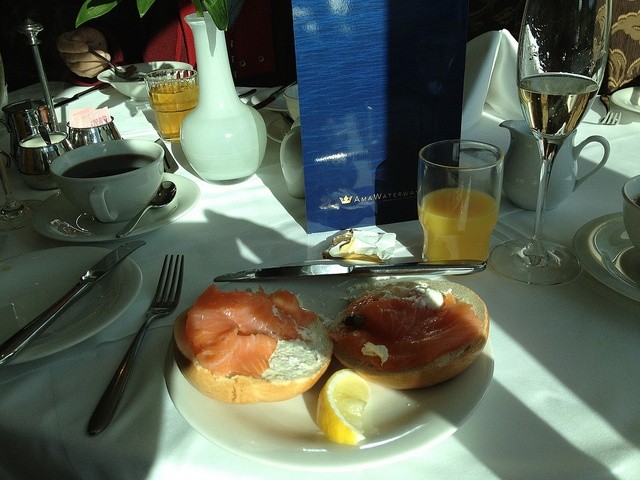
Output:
[31,172,201,243]
[611,86,640,113]
[250,85,292,111]
[163,276,496,464]
[258,108,293,144]
[571,210,640,305]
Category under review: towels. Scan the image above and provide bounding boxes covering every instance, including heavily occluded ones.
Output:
[461,28,525,148]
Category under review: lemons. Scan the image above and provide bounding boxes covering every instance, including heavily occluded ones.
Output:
[316,368,369,449]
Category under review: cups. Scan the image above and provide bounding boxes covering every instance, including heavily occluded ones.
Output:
[499,119,610,212]
[142,69,199,143]
[417,138,505,271]
[48,139,166,224]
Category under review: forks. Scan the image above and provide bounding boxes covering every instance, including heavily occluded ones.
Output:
[86,253,185,435]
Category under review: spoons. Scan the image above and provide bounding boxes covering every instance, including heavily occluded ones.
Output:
[87,47,136,77]
[115,180,177,238]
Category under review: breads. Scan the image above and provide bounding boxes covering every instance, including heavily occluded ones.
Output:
[174,285,335,404]
[335,278,490,391]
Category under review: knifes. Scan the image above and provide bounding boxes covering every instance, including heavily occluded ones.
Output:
[248,81,297,109]
[211,257,488,282]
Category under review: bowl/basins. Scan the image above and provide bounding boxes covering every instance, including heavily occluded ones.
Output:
[622,170,640,246]
[96,60,194,100]
[284,84,309,121]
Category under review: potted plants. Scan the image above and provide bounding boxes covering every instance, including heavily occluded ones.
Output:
[75,1,267,182]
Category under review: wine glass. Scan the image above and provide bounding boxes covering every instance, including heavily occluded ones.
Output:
[488,0,611,287]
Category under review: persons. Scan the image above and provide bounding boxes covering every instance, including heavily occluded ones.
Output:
[58,0,274,86]
